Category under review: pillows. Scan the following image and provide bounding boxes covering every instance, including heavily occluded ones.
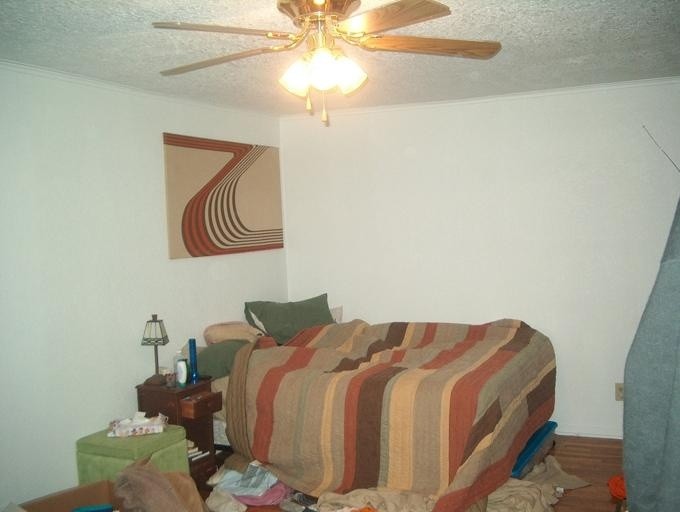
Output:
[192,293,344,381]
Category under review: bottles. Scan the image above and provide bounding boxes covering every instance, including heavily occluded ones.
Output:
[173,350,188,390]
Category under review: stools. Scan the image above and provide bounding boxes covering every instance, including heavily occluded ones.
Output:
[76,424,191,489]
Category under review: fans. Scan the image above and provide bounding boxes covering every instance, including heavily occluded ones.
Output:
[152,0,503,78]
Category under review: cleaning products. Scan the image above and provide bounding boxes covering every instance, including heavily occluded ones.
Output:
[176,361,187,388]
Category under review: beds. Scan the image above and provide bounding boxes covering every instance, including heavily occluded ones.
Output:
[174,318,559,512]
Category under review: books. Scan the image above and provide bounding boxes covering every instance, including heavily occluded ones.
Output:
[185,439,211,462]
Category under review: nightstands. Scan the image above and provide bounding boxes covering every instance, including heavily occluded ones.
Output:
[136,375,222,501]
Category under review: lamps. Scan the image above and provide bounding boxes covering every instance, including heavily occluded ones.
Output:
[282,24,371,127]
[141,314,171,387]
